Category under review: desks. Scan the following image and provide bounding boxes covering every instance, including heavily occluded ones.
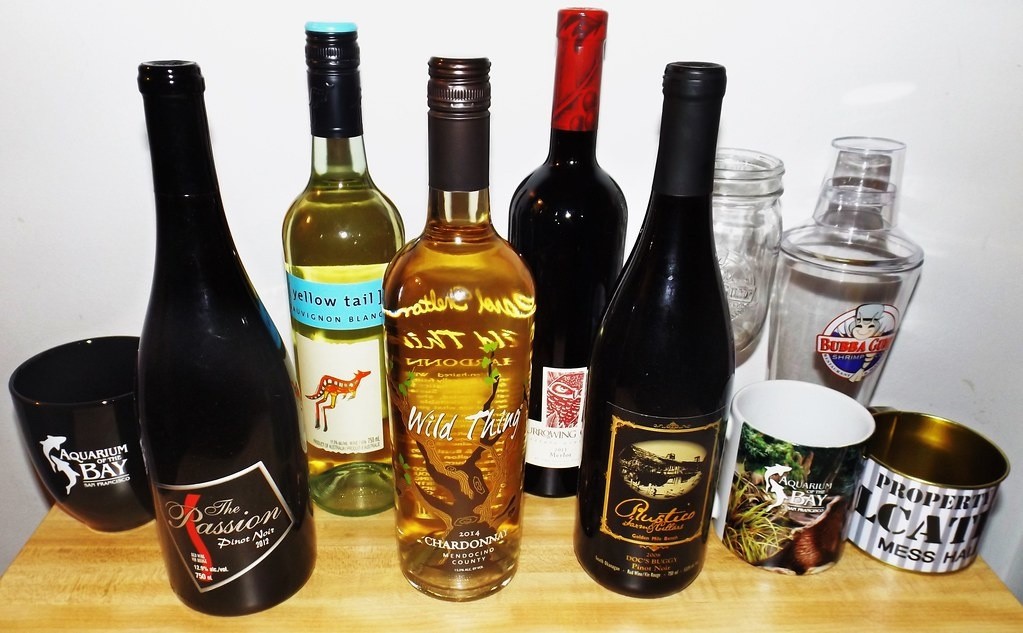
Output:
[0,491,1023,633]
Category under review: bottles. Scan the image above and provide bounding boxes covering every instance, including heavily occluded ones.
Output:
[706,147,785,353]
[574,61,734,597]
[380,53,535,603]
[281,19,407,515]
[508,6,629,500]
[767,137,925,404]
[137,60,315,615]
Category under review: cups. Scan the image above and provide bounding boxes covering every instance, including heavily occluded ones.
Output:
[847,410,1011,574]
[711,379,876,574]
[8,336,154,532]
